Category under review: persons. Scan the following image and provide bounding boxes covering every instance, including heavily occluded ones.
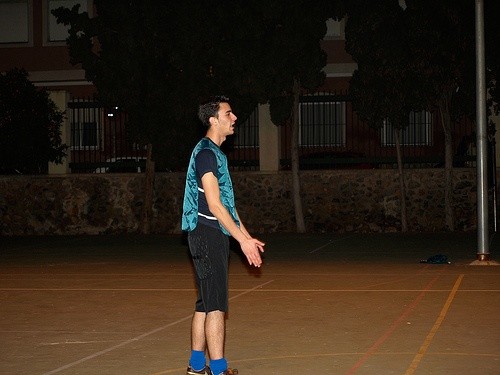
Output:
[177,92,267,375]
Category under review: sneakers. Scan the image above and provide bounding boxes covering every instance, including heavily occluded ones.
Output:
[210,368,240,375]
[187,366,212,375]
